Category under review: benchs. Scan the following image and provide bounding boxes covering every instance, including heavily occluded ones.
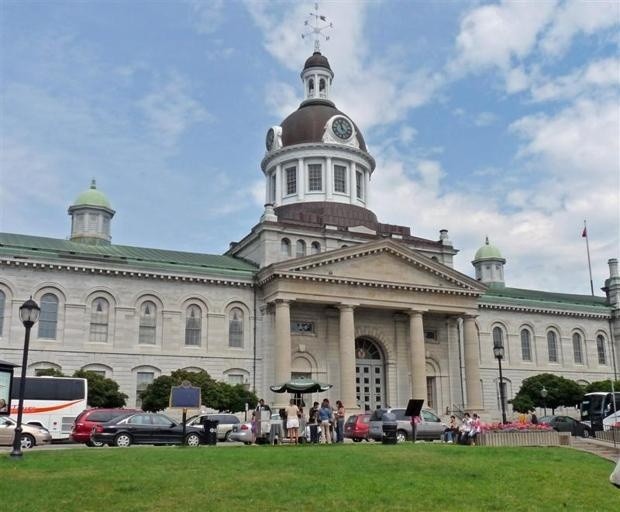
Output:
[446,431,479,444]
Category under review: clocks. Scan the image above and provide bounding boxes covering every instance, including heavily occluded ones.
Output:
[332,118,352,139]
[266,128,274,151]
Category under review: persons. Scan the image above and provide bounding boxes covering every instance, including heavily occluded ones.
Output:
[458,413,473,445]
[468,413,481,446]
[251,399,345,445]
[443,415,457,443]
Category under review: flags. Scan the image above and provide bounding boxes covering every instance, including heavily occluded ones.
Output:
[582,227,588,238]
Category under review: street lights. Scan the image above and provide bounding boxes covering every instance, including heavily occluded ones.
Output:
[8,295,41,459]
[494,341,509,426]
[540,385,549,416]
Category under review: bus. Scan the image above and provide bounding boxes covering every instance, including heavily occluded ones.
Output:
[7,374,89,441]
[580,391,620,431]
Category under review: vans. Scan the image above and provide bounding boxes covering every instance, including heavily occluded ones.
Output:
[182,413,242,442]
[368,406,451,444]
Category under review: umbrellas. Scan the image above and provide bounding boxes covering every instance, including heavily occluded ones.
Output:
[270,376,333,407]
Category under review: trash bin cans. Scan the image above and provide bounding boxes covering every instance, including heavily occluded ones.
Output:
[382,423,397,445]
[202,419,219,445]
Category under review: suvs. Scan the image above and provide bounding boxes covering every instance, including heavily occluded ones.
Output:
[69,408,146,447]
[342,415,375,442]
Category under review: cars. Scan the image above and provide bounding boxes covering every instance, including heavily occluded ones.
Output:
[230,414,337,445]
[90,411,217,446]
[0,414,53,449]
[537,415,593,438]
[603,415,620,432]
